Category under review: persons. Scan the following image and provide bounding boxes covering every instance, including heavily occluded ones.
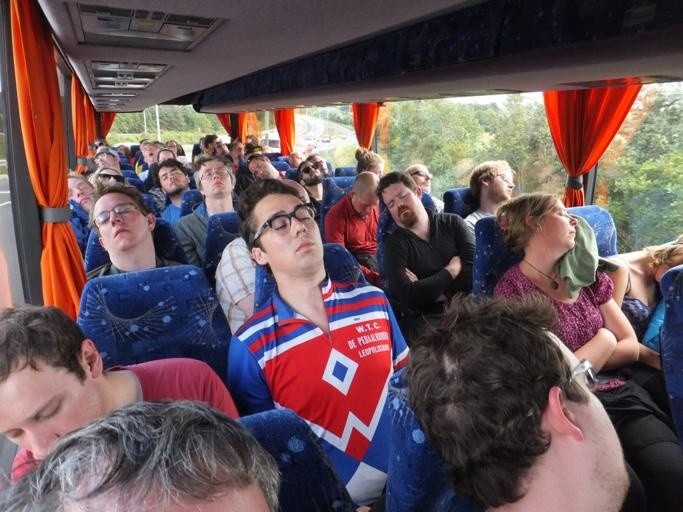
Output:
[1,134,683,512]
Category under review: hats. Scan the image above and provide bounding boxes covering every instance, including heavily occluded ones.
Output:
[412,170,427,176]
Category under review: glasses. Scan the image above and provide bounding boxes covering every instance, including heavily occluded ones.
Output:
[491,170,516,184]
[92,201,145,230]
[198,168,233,183]
[314,160,324,166]
[96,173,125,186]
[555,358,602,399]
[300,164,318,178]
[249,201,319,251]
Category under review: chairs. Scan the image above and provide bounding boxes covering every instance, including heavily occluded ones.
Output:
[69,144,682,510]
